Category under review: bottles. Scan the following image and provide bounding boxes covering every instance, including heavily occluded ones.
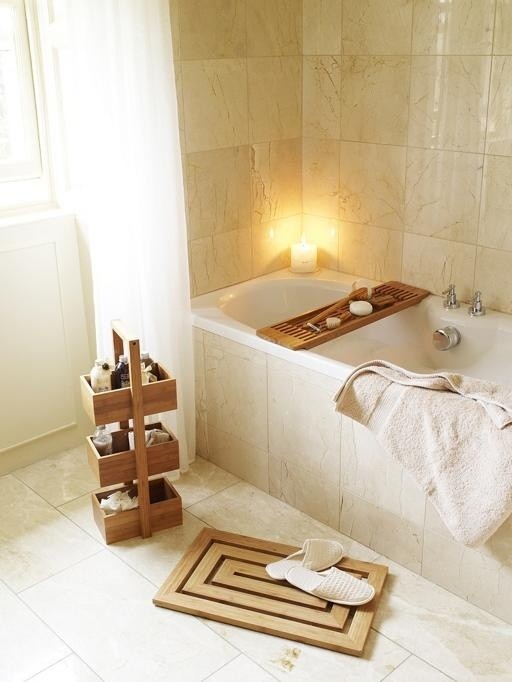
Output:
[93,424,112,455]
[114,352,154,389]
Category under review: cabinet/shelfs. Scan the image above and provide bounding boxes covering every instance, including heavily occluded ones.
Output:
[79,318,183,545]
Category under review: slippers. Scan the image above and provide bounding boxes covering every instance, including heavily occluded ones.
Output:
[266,539,376,606]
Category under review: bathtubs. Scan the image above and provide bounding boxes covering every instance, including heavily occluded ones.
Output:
[191,268,512,382]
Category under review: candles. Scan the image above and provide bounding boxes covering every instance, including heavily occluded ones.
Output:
[289,242,318,273]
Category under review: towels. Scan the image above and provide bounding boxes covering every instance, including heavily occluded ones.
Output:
[332,358,511,548]
